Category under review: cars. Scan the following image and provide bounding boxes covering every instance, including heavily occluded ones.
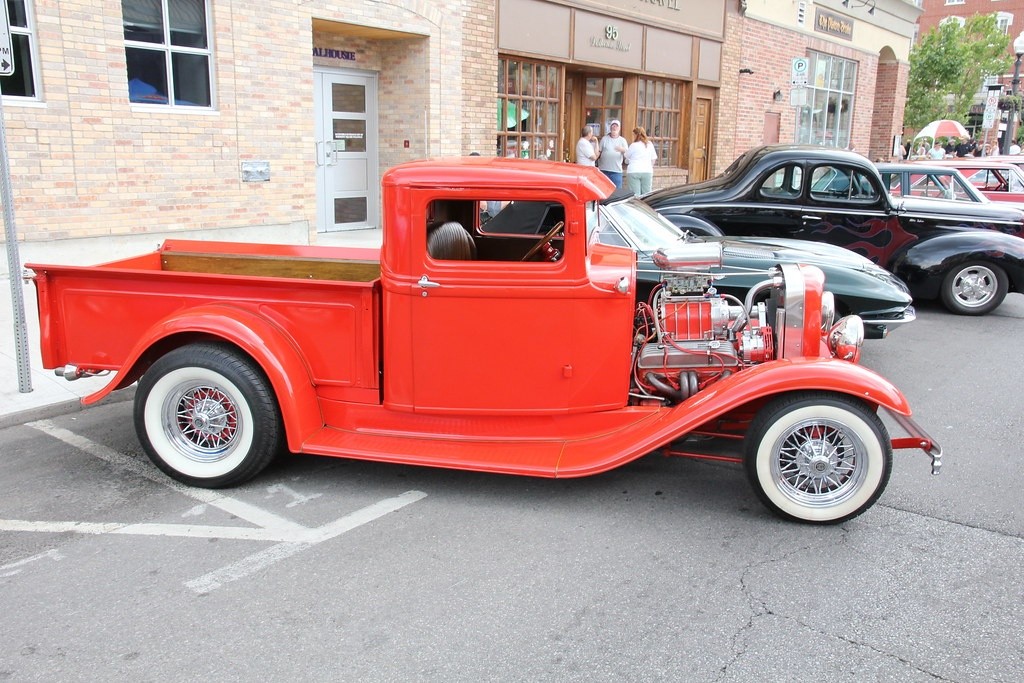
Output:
[598,144,1024,316]
[480,189,916,338]
[890,153,1024,203]
[812,161,1024,221]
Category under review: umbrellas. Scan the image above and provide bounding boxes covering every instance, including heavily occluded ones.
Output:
[913,120,970,149]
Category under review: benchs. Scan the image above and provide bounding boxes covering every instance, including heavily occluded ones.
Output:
[425,220,478,261]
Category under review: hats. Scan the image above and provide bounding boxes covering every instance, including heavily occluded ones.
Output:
[611,120,620,127]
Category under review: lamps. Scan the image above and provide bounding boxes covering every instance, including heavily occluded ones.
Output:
[851,0,876,16]
[842,0,849,8]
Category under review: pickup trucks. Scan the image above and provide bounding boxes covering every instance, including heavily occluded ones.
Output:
[23,156,945,525]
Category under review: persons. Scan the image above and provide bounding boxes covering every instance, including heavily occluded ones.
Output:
[470,152,502,227]
[876,158,884,163]
[575,124,600,167]
[598,120,629,189]
[1009,140,1021,155]
[900,137,1001,158]
[927,141,946,160]
[624,127,658,198]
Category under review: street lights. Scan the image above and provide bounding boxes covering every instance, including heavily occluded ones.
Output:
[1002,32,1024,155]
